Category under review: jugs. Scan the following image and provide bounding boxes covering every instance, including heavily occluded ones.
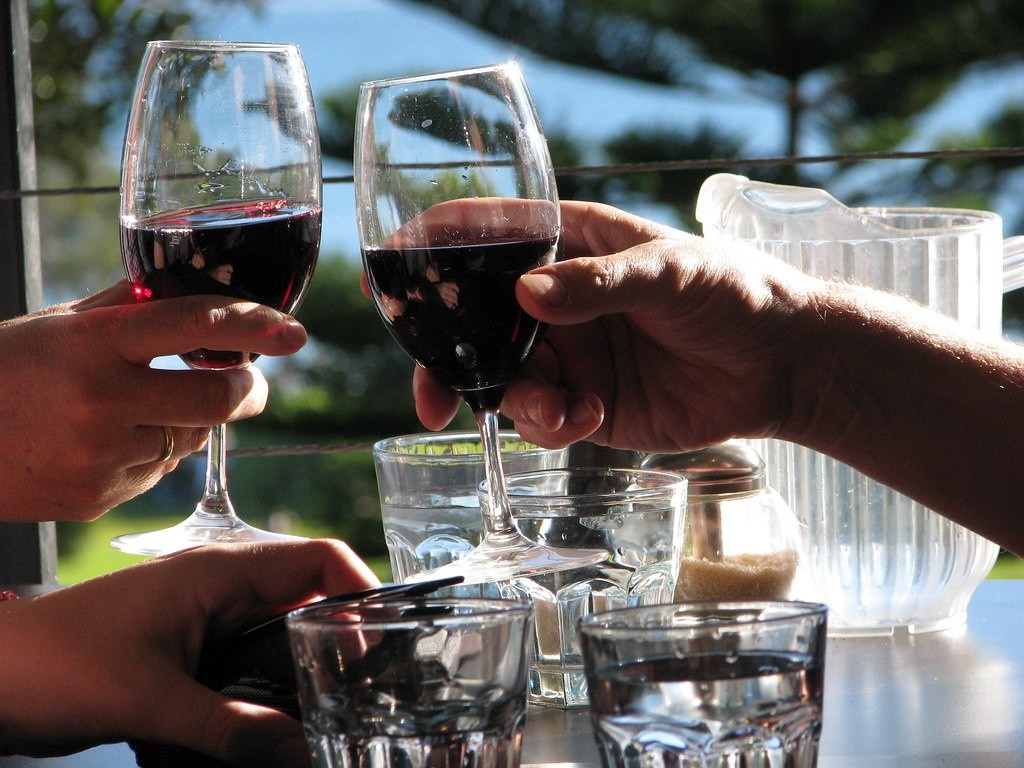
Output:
[690,171,1024,640]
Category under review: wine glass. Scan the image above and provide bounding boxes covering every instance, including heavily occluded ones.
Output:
[109,38,321,563]
[351,57,612,592]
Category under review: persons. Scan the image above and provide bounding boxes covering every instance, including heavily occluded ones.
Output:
[0,278,307,523]
[362,197,1023,561]
[1,537,403,767]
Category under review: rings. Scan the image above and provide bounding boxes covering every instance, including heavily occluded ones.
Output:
[156,425,174,462]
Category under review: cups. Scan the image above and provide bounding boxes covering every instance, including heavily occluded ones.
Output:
[285,430,829,768]
